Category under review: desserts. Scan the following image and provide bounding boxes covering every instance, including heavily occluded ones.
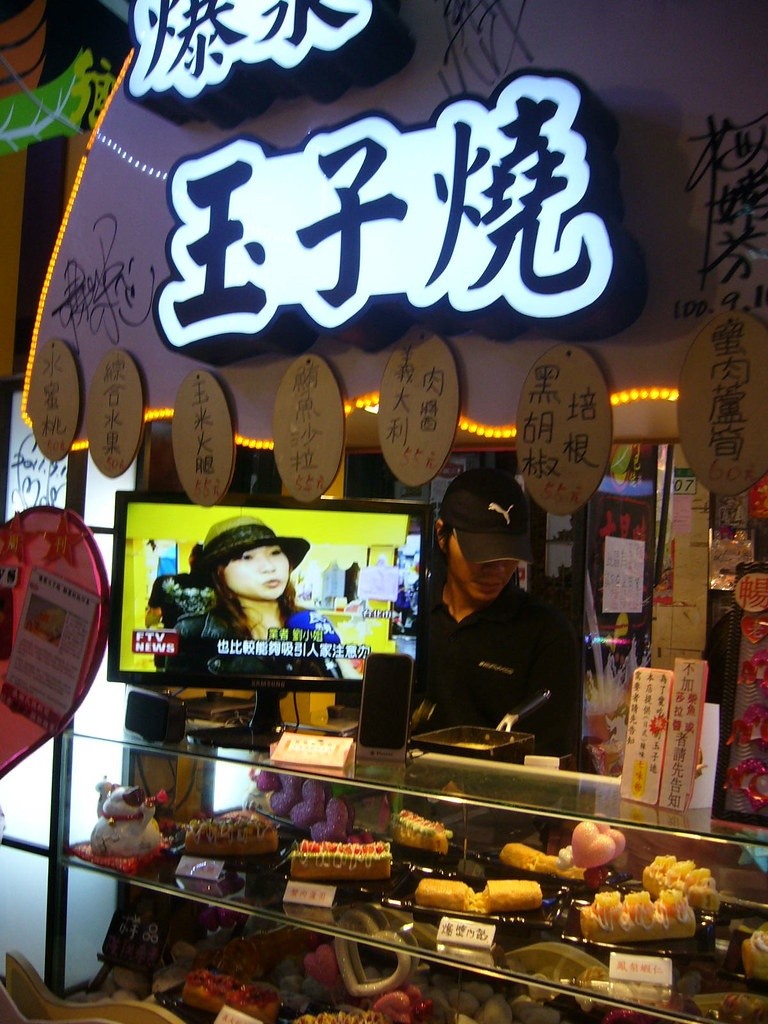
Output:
[74,809,768,1024]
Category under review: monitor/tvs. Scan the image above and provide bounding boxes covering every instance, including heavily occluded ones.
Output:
[106,490,434,740]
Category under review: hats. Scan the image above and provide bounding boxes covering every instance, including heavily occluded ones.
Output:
[439,468,534,564]
[202,516,310,574]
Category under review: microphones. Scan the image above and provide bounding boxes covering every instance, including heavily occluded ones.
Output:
[285,610,345,678]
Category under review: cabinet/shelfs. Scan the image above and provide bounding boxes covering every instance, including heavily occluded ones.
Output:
[53,725,767,1024]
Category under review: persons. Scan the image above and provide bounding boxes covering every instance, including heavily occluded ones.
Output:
[145,517,343,677]
[349,469,567,753]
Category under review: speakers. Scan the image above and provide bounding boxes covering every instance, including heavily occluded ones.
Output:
[357,654,414,764]
[124,689,187,743]
